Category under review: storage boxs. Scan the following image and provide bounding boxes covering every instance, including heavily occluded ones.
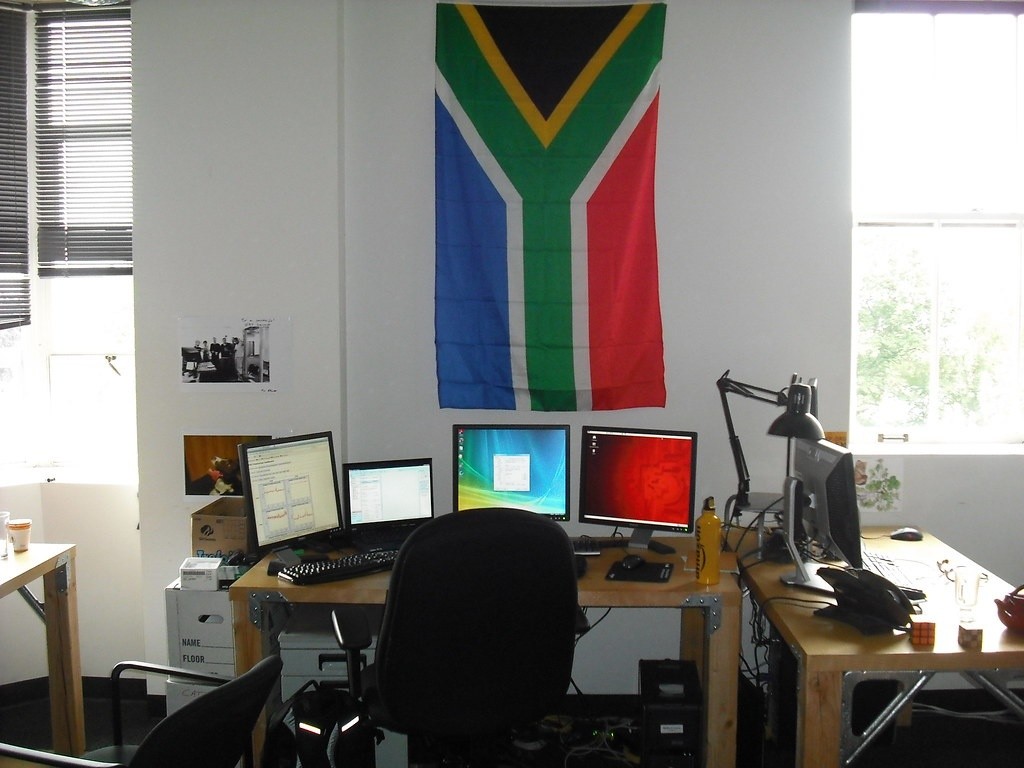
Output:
[179,557,222,592]
[219,565,252,590]
[191,496,248,566]
[165,577,236,686]
[165,680,221,718]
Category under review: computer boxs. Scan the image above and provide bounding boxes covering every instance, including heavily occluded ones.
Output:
[769,624,901,747]
[638,659,704,768]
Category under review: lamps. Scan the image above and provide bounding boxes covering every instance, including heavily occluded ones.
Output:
[715,369,826,553]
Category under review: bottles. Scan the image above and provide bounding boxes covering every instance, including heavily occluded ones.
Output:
[695,496,722,585]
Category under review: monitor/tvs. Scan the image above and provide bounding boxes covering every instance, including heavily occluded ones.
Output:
[578,425,698,554]
[779,432,864,593]
[452,424,571,523]
[237,430,345,576]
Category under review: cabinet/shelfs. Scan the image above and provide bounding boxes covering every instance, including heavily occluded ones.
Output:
[279,602,408,768]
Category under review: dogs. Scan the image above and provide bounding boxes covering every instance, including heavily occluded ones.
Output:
[208,455,242,495]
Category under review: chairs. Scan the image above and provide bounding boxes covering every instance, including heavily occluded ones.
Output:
[0,654,284,768]
[293,515,591,768]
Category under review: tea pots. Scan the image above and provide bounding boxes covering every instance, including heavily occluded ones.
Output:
[994,584,1024,631]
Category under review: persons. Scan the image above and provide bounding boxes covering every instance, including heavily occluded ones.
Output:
[193,336,244,362]
[188,457,239,496]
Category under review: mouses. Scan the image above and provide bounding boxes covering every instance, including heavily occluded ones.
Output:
[622,555,645,570]
[890,527,924,541]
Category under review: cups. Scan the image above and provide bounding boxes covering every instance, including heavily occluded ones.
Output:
[951,561,981,607]
[0,511,11,559]
[6,519,32,553]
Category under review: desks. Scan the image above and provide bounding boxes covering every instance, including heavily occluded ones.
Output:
[0,540,88,757]
[724,524,1024,768]
[228,536,743,768]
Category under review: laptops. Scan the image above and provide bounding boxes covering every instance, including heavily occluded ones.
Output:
[343,457,437,553]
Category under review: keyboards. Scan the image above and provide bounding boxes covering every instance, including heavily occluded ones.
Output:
[278,549,400,585]
[860,550,927,601]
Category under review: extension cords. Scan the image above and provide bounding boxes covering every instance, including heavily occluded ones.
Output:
[572,720,626,747]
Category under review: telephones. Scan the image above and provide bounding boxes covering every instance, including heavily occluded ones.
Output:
[815,568,916,635]
